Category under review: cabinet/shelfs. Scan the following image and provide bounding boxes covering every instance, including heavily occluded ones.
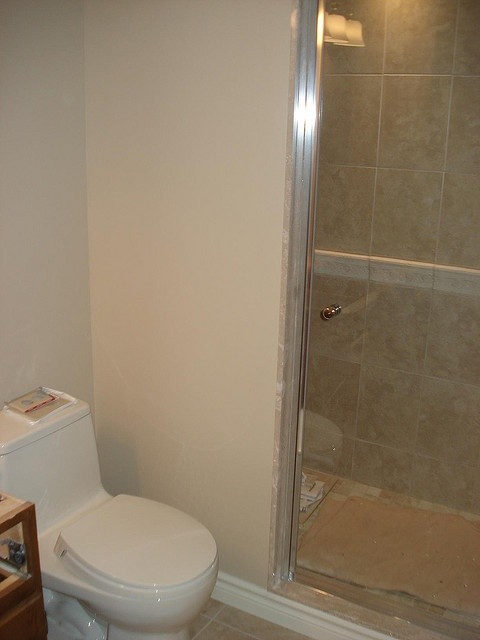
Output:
[0,494,47,639]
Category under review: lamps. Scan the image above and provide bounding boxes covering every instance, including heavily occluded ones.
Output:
[335,5,365,48]
[327,2,349,44]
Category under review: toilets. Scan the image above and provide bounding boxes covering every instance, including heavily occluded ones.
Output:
[0,386,220,640]
